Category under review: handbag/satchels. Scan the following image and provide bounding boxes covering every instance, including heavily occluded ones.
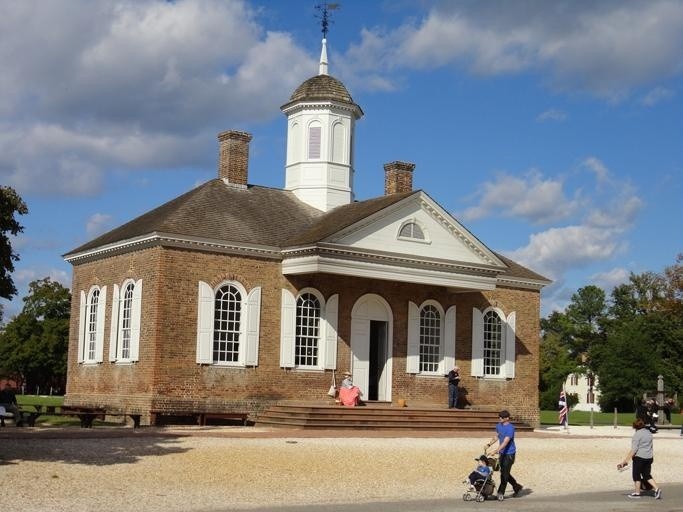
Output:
[328,386,337,397]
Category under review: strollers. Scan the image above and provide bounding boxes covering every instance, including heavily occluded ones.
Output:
[462,449,503,502]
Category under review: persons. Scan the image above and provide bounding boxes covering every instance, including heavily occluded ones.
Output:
[485,410,523,500]
[680,409,683,435]
[342,375,361,405]
[448,367,461,408]
[457,382,471,408]
[0,383,23,427]
[462,456,490,492]
[618,398,662,500]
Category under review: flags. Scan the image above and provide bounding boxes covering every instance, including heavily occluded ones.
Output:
[558,389,568,426]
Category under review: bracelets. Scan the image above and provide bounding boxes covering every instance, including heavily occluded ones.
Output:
[487,443,491,447]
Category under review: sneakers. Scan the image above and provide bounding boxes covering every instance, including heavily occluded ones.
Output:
[497,493,503,501]
[514,485,522,496]
[655,488,662,500]
[628,493,641,499]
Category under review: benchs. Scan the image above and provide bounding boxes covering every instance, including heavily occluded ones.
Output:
[0,404,250,428]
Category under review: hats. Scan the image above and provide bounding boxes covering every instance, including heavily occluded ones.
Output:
[475,455,489,464]
[499,411,510,417]
[342,372,353,375]
